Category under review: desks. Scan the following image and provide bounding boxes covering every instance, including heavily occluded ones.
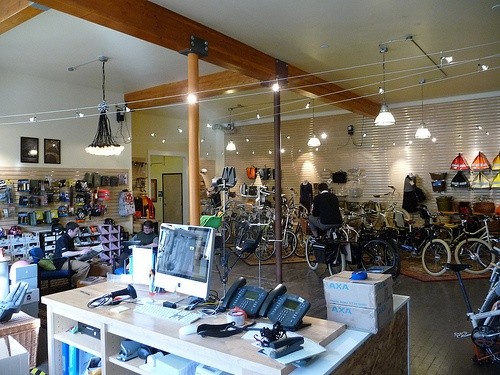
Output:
[432,210,495,216]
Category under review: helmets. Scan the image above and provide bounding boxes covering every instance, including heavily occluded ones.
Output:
[52,224,63,235]
[0,228,3,238]
[9,226,21,236]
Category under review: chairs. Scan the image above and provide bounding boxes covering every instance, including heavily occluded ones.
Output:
[29,247,75,296]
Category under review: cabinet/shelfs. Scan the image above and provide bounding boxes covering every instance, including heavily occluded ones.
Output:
[450,168,500,190]
[0,224,122,261]
[238,184,265,209]
[38,278,368,375]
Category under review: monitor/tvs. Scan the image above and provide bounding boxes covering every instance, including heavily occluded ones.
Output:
[153,222,218,311]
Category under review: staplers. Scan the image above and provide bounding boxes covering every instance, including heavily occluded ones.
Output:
[269,336,305,359]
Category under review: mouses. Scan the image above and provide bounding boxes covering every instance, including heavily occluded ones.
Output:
[179,324,199,336]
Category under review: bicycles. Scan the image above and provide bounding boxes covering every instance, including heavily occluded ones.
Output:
[198,185,500,375]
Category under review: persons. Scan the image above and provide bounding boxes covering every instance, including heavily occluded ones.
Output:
[53,222,90,288]
[120,220,159,270]
[308,182,343,241]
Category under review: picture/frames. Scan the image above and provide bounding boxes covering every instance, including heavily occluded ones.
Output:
[20,136,39,163]
[43,138,61,164]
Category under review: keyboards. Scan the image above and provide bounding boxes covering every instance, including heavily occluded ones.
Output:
[133,303,201,324]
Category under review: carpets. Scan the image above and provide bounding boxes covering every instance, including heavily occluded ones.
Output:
[380,245,494,283]
[229,246,369,266]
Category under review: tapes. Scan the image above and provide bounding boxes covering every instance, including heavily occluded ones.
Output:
[351,270,367,280]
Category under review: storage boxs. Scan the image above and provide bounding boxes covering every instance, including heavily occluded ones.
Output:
[323,270,394,334]
[8,264,39,304]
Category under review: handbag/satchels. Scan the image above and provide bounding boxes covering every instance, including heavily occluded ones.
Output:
[314,241,333,262]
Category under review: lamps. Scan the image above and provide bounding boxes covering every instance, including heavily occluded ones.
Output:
[415,79,430,141]
[226,107,237,150]
[375,45,396,126]
[307,103,320,146]
[83,56,125,156]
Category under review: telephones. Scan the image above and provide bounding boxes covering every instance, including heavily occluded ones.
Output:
[259,283,311,330]
[219,276,269,319]
